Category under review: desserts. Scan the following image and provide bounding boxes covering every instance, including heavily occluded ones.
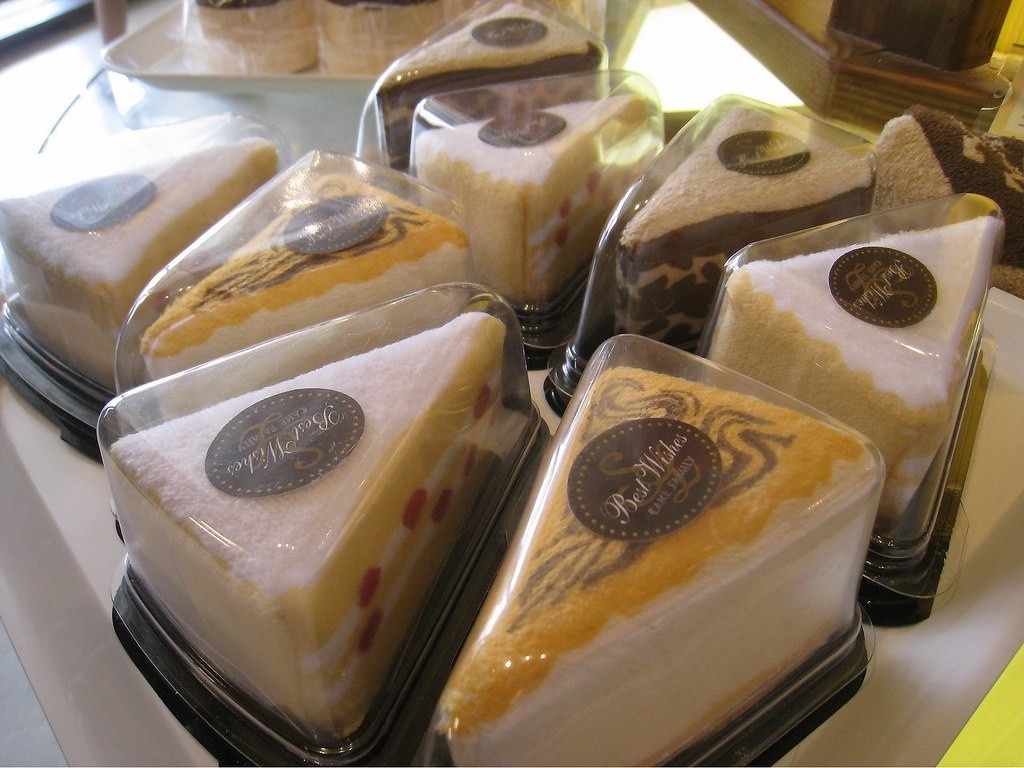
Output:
[0,0,999,768]
